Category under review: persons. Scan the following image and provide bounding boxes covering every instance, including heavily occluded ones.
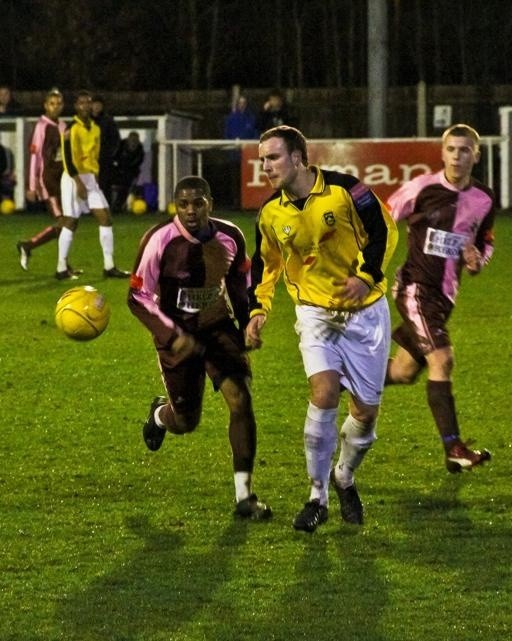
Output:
[118,133,143,201]
[55,93,129,280]
[385,125,494,474]
[230,96,257,174]
[89,97,123,211]
[127,177,273,523]
[242,127,394,532]
[263,93,296,132]
[0,88,15,213]
[15,89,64,272]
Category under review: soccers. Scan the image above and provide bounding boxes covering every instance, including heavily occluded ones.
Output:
[54,284,111,341]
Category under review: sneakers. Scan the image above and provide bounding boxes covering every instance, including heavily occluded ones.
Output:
[237,494,271,521]
[446,443,491,473]
[292,499,329,530]
[68,265,84,274]
[18,240,31,272]
[55,269,80,280]
[104,267,131,279]
[332,469,364,525]
[143,395,168,451]
